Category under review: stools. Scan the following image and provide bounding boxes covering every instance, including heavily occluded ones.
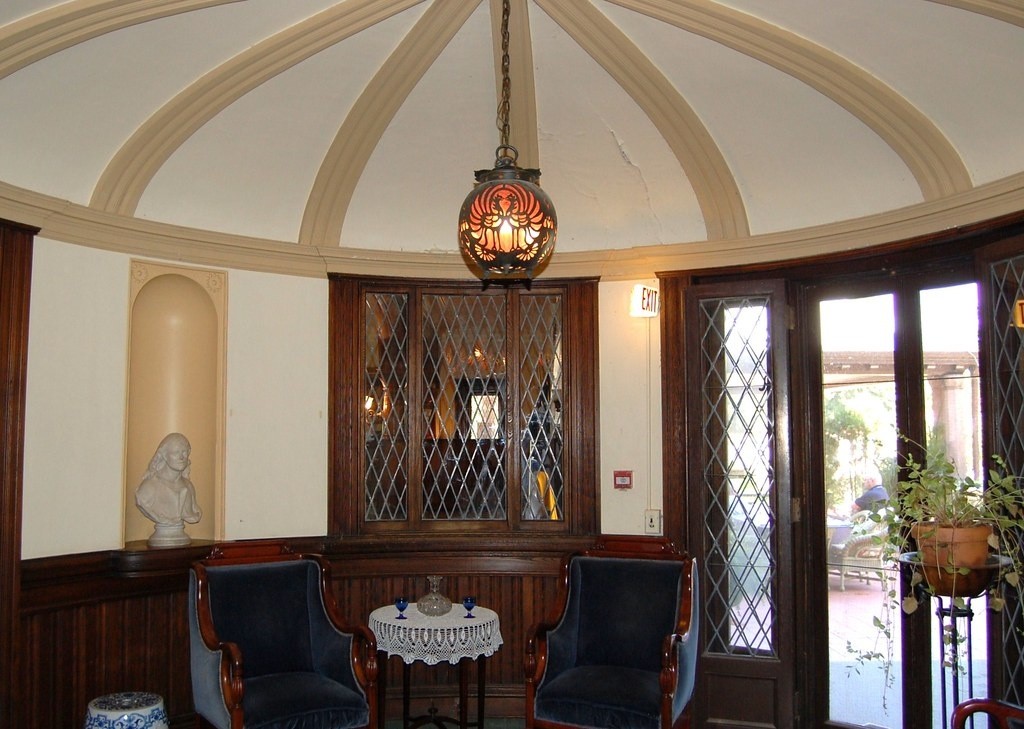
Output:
[84,691,171,729]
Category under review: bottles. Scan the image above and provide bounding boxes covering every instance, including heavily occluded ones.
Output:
[417,576,452,617]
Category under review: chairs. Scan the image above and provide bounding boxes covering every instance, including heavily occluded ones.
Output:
[838,504,899,591]
[523,535,701,729]
[189,539,379,729]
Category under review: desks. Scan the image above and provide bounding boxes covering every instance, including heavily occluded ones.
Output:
[736,514,854,550]
[369,602,505,729]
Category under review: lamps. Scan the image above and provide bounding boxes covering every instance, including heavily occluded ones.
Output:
[457,0,557,281]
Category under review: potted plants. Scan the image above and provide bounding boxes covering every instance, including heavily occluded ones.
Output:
[848,422,1024,714]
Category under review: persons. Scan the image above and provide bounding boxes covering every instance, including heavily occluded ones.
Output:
[851,470,890,514]
[137,433,203,525]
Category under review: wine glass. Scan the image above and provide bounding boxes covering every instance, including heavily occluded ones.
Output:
[395,597,408,619]
[462,596,476,618]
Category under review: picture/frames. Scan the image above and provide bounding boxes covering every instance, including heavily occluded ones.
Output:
[455,378,507,440]
